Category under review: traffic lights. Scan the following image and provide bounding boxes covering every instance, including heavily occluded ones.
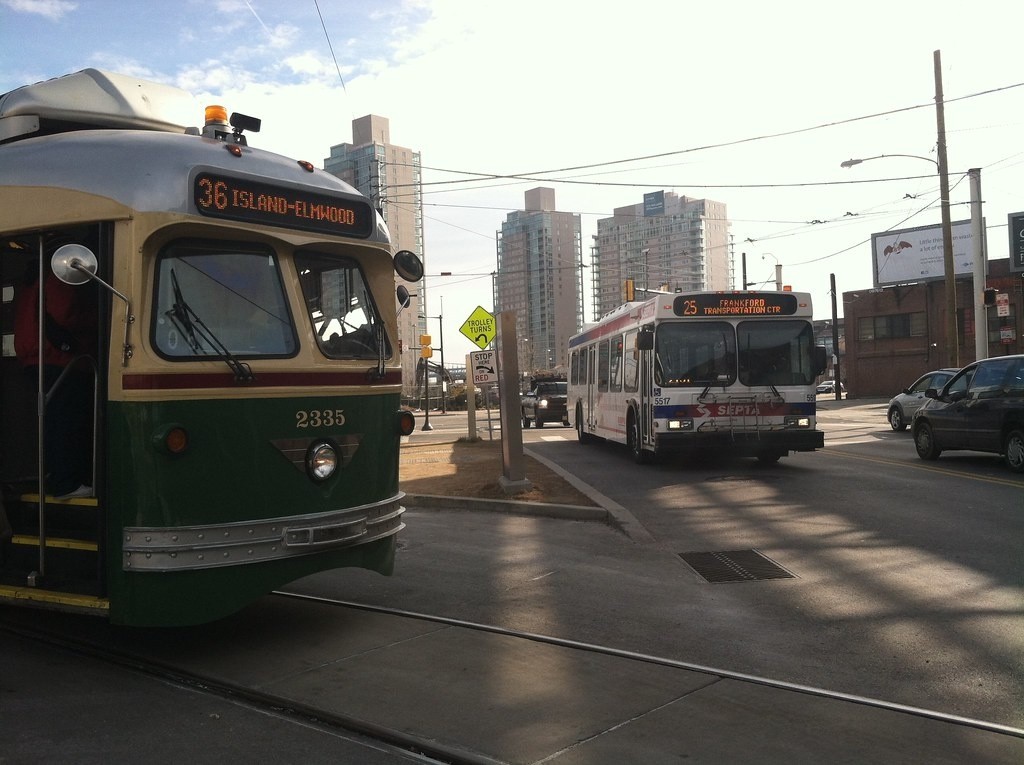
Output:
[674,287,683,293]
[622,280,635,302]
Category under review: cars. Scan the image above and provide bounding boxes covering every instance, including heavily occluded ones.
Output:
[816,379,845,394]
[886,366,975,433]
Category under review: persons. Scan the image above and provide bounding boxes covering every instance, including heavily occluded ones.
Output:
[14,232,93,501]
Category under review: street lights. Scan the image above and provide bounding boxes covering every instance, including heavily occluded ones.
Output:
[746,264,783,291]
[411,323,418,386]
[418,314,446,414]
[840,153,958,367]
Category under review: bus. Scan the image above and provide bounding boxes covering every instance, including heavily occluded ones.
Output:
[0,61,425,630]
[567,283,827,465]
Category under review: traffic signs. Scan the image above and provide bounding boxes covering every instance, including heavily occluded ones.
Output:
[458,306,496,350]
[469,348,500,385]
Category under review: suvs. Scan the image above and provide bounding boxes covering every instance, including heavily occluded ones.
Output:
[519,382,571,430]
[909,354,1024,477]
